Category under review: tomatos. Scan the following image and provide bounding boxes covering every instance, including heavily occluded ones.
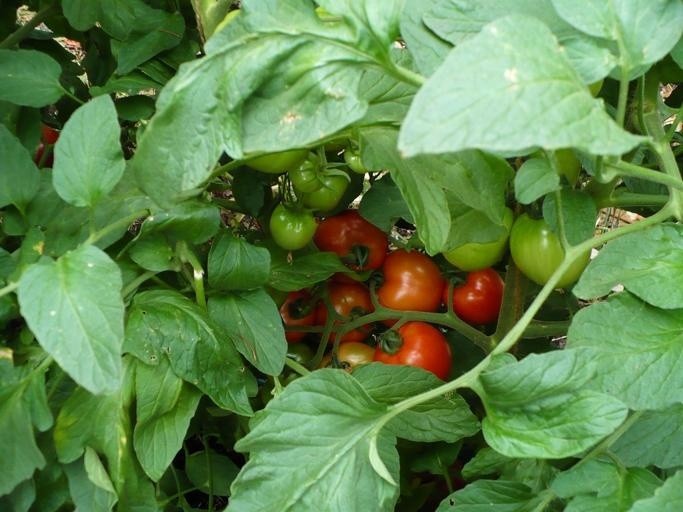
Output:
[31,0,604,383]
